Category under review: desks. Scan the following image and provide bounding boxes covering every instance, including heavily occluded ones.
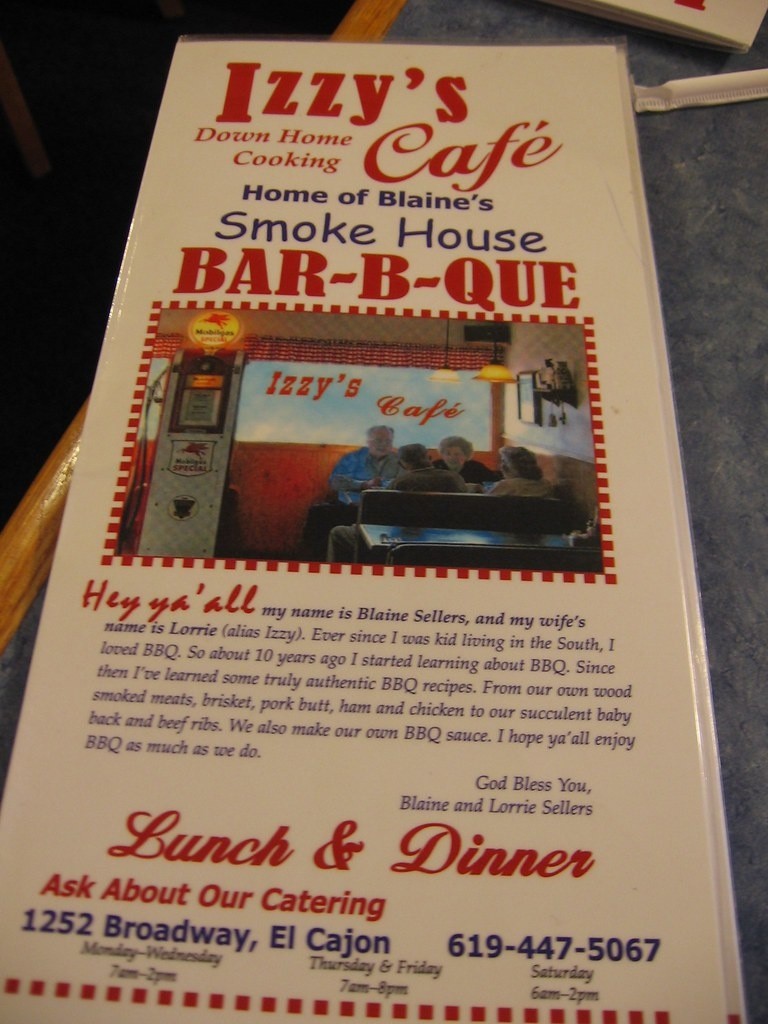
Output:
[1,0,768,1024]
[355,526,602,570]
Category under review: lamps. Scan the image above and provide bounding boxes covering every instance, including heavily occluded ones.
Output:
[428,319,459,382]
[472,338,516,383]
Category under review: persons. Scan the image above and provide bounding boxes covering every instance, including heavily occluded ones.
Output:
[488,445,557,499]
[329,425,403,521]
[326,445,467,566]
[432,436,502,482]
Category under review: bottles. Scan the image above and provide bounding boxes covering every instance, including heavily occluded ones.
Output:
[544,358,554,389]
[554,361,571,389]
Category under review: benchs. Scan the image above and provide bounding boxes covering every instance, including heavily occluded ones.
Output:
[356,489,565,532]
[385,542,604,573]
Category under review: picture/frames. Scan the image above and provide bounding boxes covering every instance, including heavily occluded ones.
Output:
[516,371,541,425]
